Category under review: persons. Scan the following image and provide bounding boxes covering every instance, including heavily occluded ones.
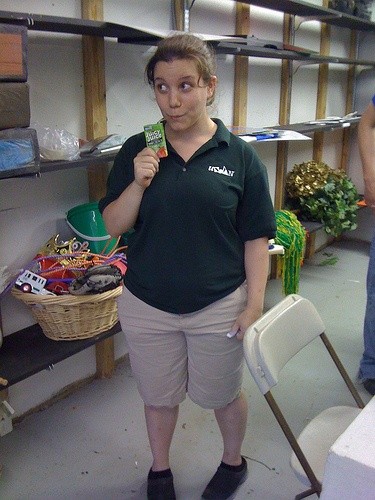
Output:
[97,34,280,500]
[357,94,375,395]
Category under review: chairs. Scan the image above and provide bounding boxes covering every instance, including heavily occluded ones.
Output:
[242,294,366,500]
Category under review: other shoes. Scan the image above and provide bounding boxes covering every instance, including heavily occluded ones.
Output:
[359,370,375,396]
[148,467,177,500]
[202,456,248,500]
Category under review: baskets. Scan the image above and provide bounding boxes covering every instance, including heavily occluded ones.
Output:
[10,284,123,342]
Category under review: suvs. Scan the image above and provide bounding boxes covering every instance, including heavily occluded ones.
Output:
[15,270,57,296]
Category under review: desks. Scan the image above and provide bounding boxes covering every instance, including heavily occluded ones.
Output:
[319,396,375,500]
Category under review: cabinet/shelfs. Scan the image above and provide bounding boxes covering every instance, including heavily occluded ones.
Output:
[0,0,375,437]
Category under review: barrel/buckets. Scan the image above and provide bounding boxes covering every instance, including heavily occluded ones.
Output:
[64,201,120,260]
